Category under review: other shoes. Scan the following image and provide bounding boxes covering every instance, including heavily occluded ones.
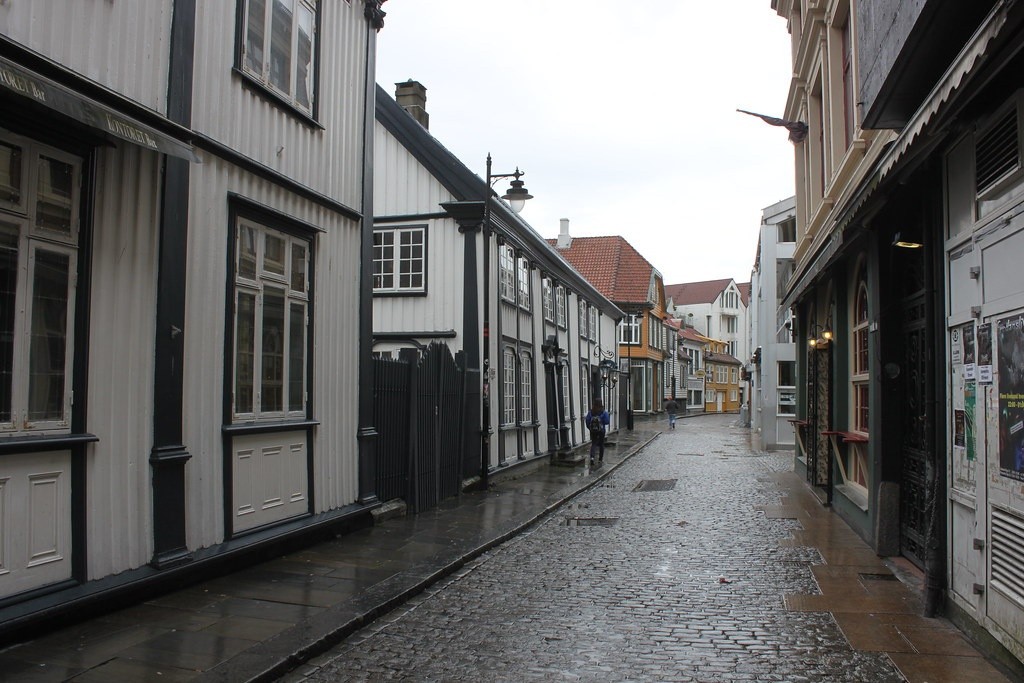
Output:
[590,457,595,466]
[598,460,603,468]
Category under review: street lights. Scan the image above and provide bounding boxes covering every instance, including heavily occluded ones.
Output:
[478,150,534,493]
[703,347,714,413]
[672,333,687,402]
[627,299,645,430]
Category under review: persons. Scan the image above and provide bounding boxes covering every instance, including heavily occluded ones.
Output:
[586,396,610,465]
[665,395,679,429]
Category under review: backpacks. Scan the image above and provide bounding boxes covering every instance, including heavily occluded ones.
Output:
[589,411,605,433]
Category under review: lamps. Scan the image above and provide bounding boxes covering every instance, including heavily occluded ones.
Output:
[600,374,608,388]
[890,225,925,248]
[593,345,618,369]
[605,376,619,389]
[807,324,822,349]
[822,314,833,340]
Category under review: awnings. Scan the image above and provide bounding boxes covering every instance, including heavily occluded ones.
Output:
[777,229,870,320]
[0,55,201,165]
[829,0,1024,241]
